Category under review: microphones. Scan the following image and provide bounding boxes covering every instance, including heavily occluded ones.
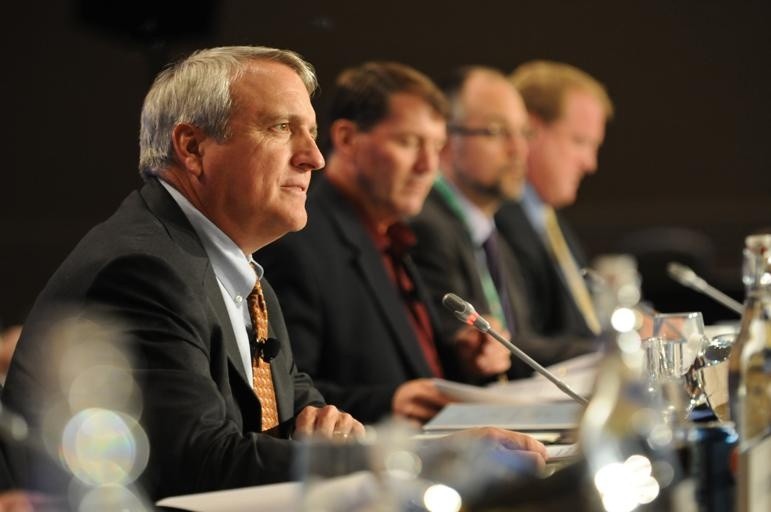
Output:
[257,336,282,363]
[668,262,744,315]
[441,293,590,407]
[583,268,689,342]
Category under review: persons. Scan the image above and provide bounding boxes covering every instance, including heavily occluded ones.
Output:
[506,55,685,381]
[256,59,515,431]
[2,44,554,512]
[402,62,541,389]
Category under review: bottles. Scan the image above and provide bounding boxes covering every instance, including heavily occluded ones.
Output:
[726,232,771,442]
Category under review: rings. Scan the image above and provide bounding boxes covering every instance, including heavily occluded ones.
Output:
[333,431,349,439]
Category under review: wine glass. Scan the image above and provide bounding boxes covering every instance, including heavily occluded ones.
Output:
[645,311,714,454]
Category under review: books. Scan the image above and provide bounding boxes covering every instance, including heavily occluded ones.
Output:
[422,396,581,433]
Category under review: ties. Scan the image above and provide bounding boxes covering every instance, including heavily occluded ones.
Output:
[544,204,599,336]
[245,262,283,440]
